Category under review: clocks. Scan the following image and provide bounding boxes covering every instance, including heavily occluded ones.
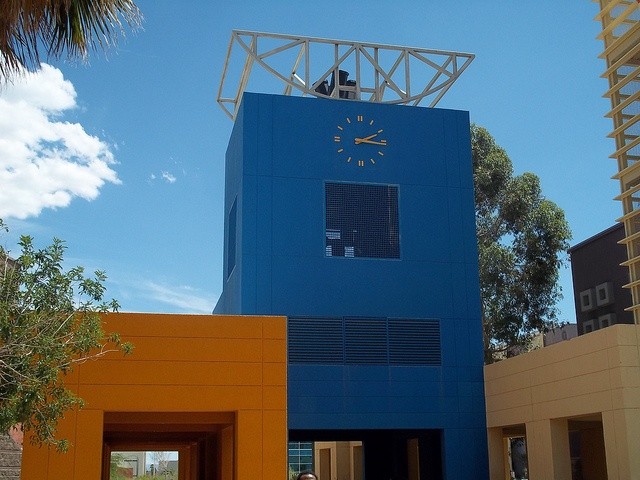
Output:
[332,114,388,167]
